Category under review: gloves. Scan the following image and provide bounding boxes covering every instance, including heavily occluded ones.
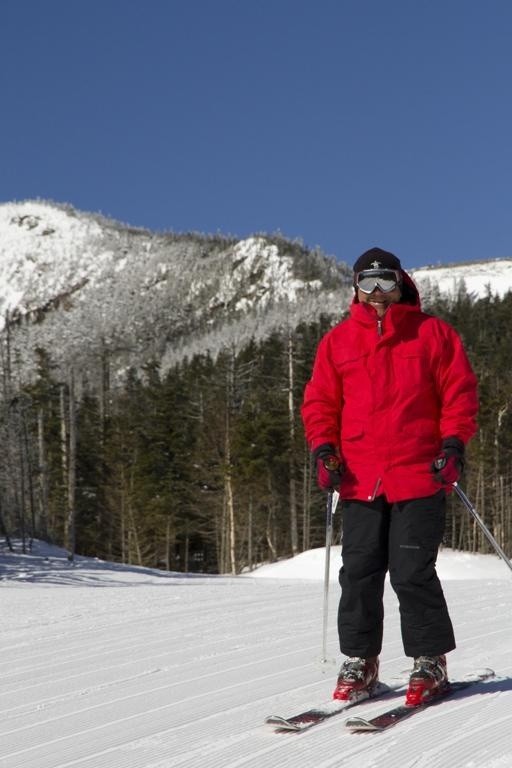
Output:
[313,443,344,493]
[428,438,464,484]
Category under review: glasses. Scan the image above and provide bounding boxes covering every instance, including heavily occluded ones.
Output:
[355,268,401,294]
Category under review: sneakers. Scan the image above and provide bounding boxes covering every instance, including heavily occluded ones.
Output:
[334,657,379,699]
[405,654,448,705]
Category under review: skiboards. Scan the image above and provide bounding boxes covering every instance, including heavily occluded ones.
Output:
[263,667,497,733]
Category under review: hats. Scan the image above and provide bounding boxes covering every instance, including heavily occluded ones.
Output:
[353,248,400,288]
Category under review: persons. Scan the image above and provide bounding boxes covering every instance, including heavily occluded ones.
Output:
[301,247,480,706]
